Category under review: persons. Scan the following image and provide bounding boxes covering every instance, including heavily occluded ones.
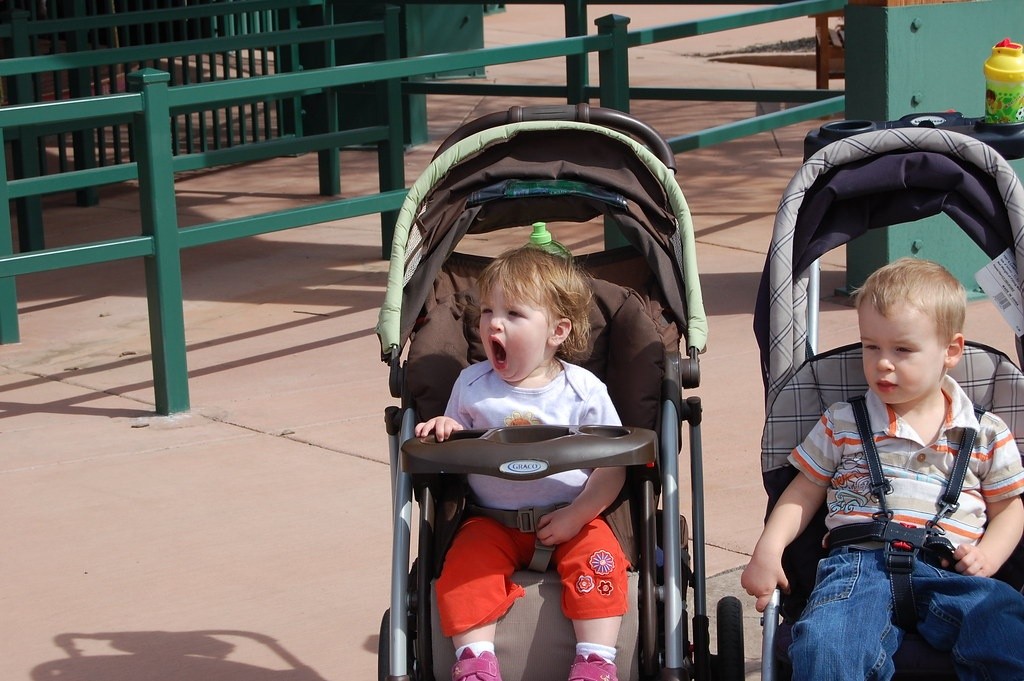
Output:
[742,256,1024,681]
[415,249,630,681]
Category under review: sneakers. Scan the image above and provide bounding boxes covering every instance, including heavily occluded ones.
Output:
[568,653,619,681]
[451,647,503,681]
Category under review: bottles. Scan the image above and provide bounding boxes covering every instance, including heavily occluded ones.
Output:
[523,222,575,264]
[983,37,1024,125]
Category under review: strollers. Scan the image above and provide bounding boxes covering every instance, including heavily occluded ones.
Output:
[378,102,746,681]
[753,112,1024,681]
